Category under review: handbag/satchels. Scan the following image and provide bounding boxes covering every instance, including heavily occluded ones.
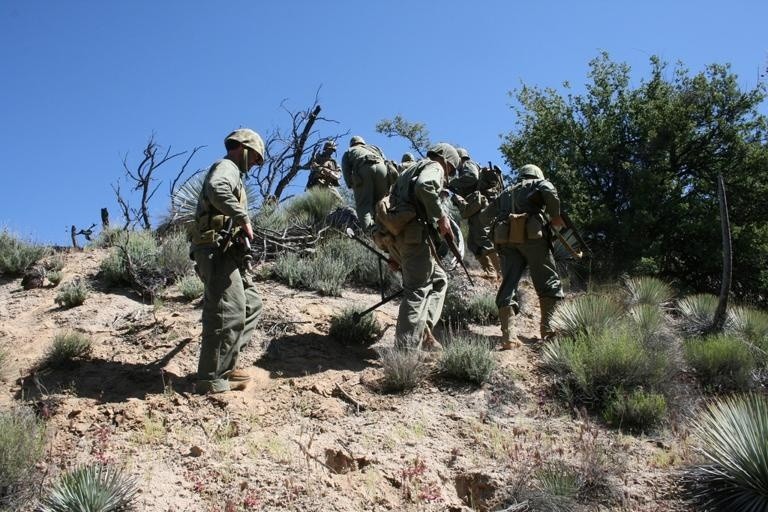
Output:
[458,190,482,219]
[376,195,417,237]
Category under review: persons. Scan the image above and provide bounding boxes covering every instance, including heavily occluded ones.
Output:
[186,127,265,395]
[375,142,462,362]
[478,164,567,351]
[304,135,505,281]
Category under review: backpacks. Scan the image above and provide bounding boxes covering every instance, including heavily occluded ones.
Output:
[466,161,505,213]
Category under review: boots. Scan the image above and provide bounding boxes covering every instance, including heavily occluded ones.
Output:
[499,306,524,350]
[486,251,504,287]
[538,296,556,339]
[475,253,499,284]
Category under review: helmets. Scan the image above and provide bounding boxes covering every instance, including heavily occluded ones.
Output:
[323,136,545,180]
[224,128,265,166]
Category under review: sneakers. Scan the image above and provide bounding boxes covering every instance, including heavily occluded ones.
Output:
[223,368,250,380]
[195,378,248,394]
[421,328,444,353]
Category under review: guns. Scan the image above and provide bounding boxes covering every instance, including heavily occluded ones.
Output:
[408,192,475,289]
[527,187,595,260]
[238,230,253,271]
